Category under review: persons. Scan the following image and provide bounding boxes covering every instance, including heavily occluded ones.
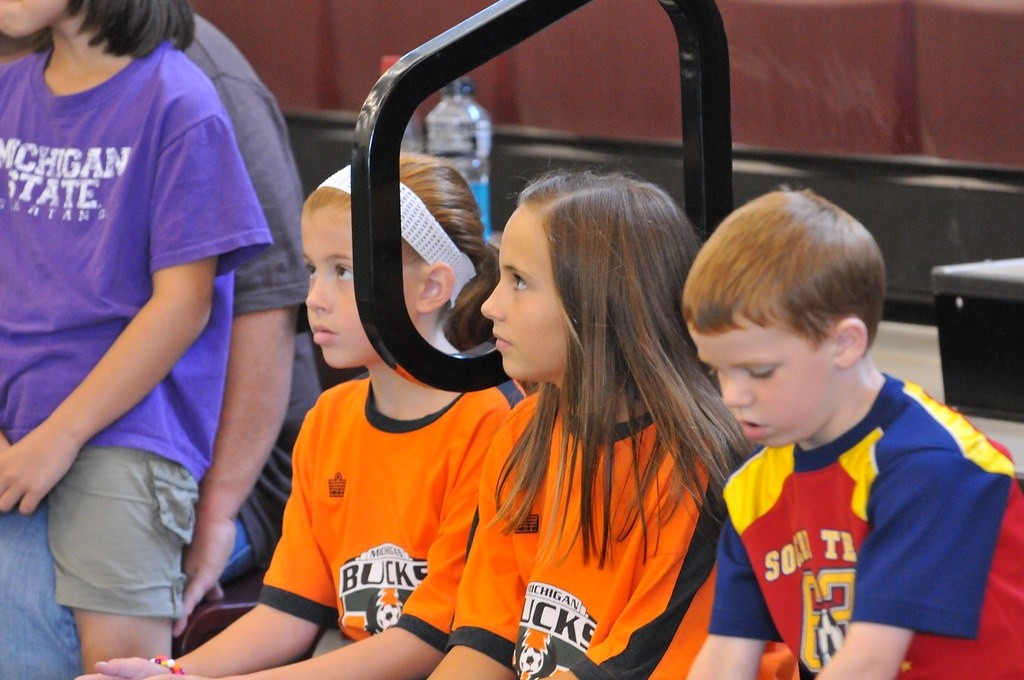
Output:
[68,150,546,680]
[681,188,1023,679]
[425,165,801,680]
[0,1,325,679]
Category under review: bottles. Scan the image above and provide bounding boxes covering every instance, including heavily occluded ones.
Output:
[380,54,420,159]
[425,79,491,249]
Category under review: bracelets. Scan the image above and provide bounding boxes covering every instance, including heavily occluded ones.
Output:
[146,654,185,676]
